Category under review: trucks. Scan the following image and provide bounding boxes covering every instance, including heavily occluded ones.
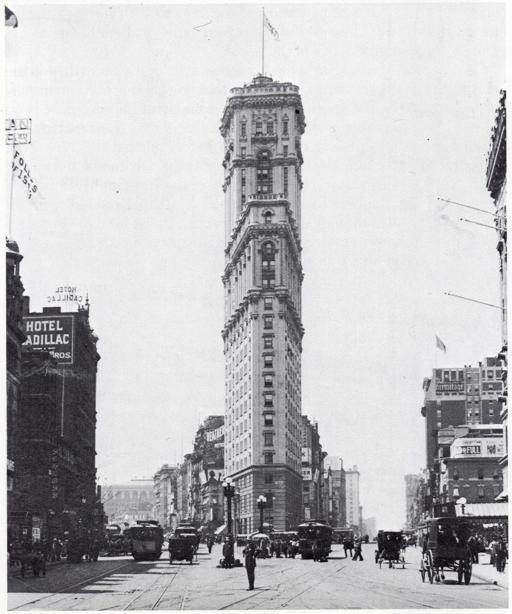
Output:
[173,526,200,550]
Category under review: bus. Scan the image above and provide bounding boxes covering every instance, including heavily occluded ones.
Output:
[128,523,163,560]
[297,521,333,560]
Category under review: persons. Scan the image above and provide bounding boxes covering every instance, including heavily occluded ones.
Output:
[342,534,354,557]
[312,536,320,560]
[223,539,232,568]
[254,529,302,560]
[207,535,213,553]
[208,470,222,482]
[8,527,126,579]
[351,539,364,561]
[466,530,508,572]
[242,540,257,591]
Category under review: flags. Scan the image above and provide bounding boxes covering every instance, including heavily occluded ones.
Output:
[5,5,17,27]
[435,336,448,352]
[12,146,40,202]
[263,14,280,38]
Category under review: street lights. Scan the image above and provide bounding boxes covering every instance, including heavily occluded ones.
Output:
[459,497,467,514]
[220,476,238,565]
[256,494,267,532]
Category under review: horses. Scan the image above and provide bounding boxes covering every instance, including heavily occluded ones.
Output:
[422,532,445,582]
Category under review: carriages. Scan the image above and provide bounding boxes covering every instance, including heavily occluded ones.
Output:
[419,515,479,585]
[374,530,406,568]
